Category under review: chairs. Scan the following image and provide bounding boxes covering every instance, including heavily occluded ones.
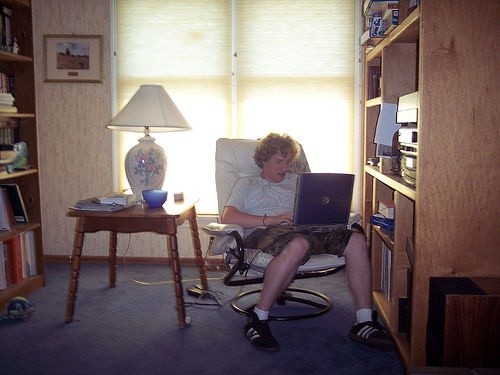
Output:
[199,137,368,321]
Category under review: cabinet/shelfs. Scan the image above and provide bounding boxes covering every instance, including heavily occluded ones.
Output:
[358,0,500,375]
[0,0,47,315]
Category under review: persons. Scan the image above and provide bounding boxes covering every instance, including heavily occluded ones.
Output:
[220,133,395,354]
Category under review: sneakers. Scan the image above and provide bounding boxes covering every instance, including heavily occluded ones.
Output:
[245,311,279,350]
[348,321,395,350]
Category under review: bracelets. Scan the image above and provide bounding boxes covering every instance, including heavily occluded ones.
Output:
[263,214,267,226]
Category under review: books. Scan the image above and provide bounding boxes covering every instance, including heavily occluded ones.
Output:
[0,231,37,290]
[378,199,394,219]
[0,73,18,112]
[0,128,15,144]
[0,7,12,46]
[380,243,391,302]
[0,183,29,231]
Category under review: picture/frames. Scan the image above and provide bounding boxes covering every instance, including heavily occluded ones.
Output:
[42,33,104,84]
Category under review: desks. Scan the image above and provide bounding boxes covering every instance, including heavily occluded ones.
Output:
[62,196,210,332]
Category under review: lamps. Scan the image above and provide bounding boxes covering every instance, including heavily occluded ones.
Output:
[103,84,192,204]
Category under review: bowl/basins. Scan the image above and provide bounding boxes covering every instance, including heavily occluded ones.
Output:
[142,190,167,207]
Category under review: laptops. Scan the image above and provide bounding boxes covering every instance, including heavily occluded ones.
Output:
[282,173,355,231]
